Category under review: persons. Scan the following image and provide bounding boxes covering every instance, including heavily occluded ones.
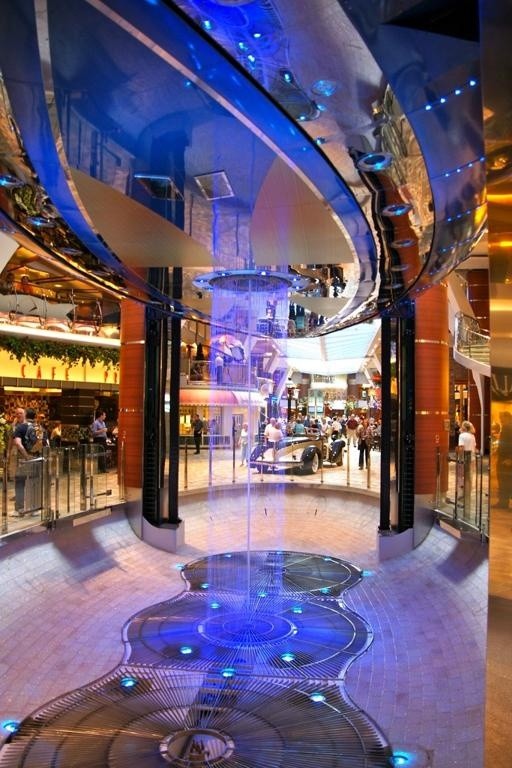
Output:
[0,403,118,517]
[238,401,382,468]
[494,410,512,508]
[491,421,499,493]
[455,421,477,501]
[186,271,339,386]
[190,413,203,454]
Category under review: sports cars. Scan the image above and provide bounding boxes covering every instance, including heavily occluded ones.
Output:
[249,428,346,474]
